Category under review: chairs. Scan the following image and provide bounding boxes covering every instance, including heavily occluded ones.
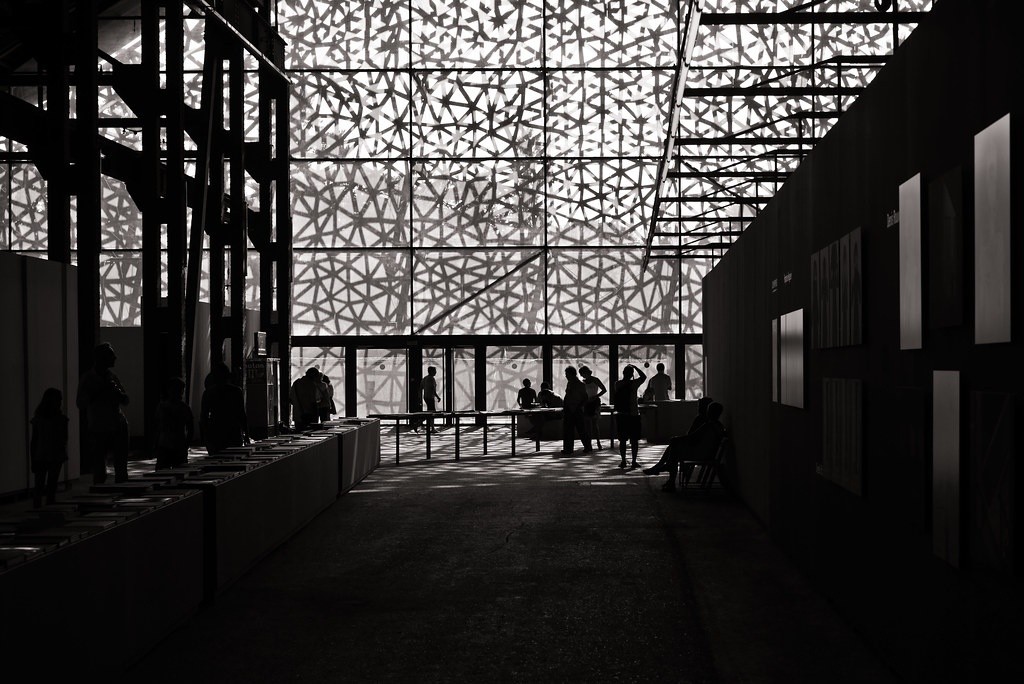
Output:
[679,437,728,491]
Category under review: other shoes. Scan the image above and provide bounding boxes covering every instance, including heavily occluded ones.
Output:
[618,462,626,467]
[643,468,659,475]
[631,462,641,468]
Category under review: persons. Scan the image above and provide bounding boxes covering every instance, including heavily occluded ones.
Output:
[650,363,672,401]
[75,341,131,490]
[612,364,647,468]
[535,382,555,408]
[30,387,69,511]
[643,383,656,402]
[145,376,195,475]
[560,366,593,454]
[578,366,606,451]
[642,396,724,490]
[517,378,536,410]
[289,366,336,430]
[412,366,441,433]
[197,360,250,457]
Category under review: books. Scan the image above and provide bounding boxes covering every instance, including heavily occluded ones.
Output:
[0,416,375,573]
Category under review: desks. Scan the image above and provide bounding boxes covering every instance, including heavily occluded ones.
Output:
[180,435,339,600]
[0,490,201,684]
[518,405,644,439]
[303,417,380,495]
